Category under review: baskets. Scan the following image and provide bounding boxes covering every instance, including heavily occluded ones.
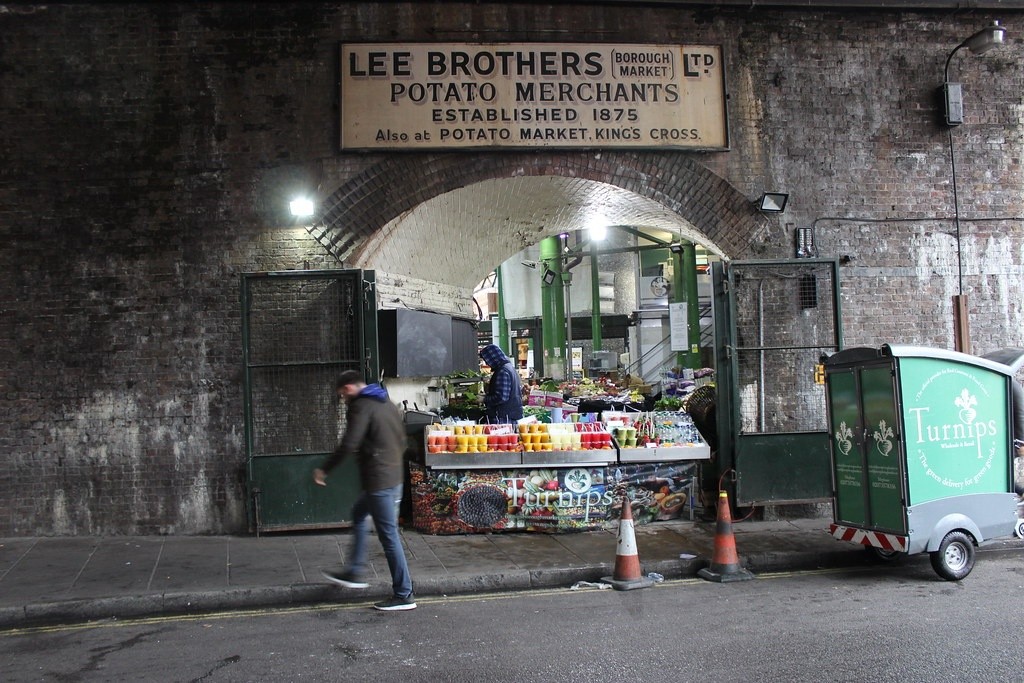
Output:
[687,386,717,430]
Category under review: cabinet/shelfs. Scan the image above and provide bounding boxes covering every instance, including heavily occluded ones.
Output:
[522,422,617,466]
[603,411,710,462]
[424,423,522,466]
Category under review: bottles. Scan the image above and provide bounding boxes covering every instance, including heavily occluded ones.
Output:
[654,412,693,428]
[658,424,699,443]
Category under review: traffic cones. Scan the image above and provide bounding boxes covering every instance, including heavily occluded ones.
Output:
[599,496,655,591]
[696,490,755,582]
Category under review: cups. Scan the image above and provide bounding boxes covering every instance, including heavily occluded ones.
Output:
[551,408,562,423]
[520,423,612,450]
[427,423,519,452]
[570,413,580,422]
[611,416,638,447]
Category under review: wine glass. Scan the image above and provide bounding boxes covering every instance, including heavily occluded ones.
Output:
[659,367,672,385]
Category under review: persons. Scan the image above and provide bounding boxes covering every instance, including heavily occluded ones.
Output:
[310,369,417,610]
[470,344,522,425]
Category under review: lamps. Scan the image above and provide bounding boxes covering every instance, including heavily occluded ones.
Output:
[670,243,684,257]
[542,269,556,285]
[754,192,789,214]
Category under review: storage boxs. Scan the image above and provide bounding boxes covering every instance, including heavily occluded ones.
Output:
[546,391,563,407]
[683,368,694,379]
[528,386,546,406]
[638,385,651,394]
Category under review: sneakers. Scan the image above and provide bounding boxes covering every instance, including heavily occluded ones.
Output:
[374,592,417,610]
[320,569,368,589]
